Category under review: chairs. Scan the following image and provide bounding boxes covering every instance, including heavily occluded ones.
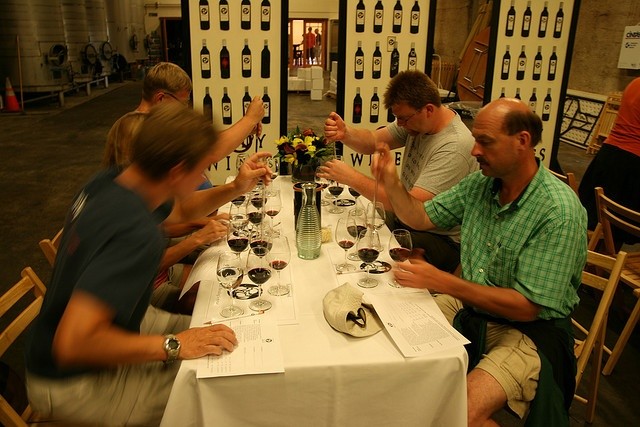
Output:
[0,265,49,427]
[38,225,63,266]
[593,184,640,377]
[499,250,628,426]
[544,166,605,251]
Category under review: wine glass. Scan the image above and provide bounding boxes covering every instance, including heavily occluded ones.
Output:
[266,235,291,295]
[388,229,413,288]
[235,153,249,174]
[251,189,267,229]
[324,155,342,199]
[266,157,280,197]
[347,208,367,261]
[348,187,363,216]
[227,220,249,274]
[365,202,386,252]
[328,180,345,214]
[229,201,250,237]
[246,247,272,312]
[246,197,266,240]
[216,252,244,319]
[264,190,282,238]
[249,226,272,267]
[356,229,380,288]
[314,166,331,206]
[335,217,358,273]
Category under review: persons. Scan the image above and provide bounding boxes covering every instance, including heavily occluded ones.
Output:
[105,60,269,217]
[576,75,640,247]
[317,70,484,268]
[298,34,305,48]
[315,27,322,66]
[96,110,232,311]
[370,97,590,426]
[24,103,276,427]
[304,26,316,64]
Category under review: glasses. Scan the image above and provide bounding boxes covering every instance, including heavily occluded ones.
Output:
[389,113,417,123]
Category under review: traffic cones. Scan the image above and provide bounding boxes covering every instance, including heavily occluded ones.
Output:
[4,76,20,114]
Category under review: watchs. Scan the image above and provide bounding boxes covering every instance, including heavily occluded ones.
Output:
[162,330,183,362]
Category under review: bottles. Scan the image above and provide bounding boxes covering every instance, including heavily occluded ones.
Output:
[261,0,271,30]
[542,88,552,121]
[514,88,521,100]
[261,39,270,78]
[393,0,402,33]
[390,42,400,78]
[410,0,420,33]
[387,108,395,123]
[242,86,252,117]
[537,2,549,38]
[295,184,322,260]
[222,87,232,124]
[203,87,213,124]
[548,45,557,80]
[373,0,383,33]
[356,0,365,32]
[408,42,417,71]
[241,0,251,30]
[200,39,211,78]
[505,0,515,36]
[528,88,536,114]
[533,45,543,80]
[516,45,527,80]
[521,0,532,37]
[553,2,564,38]
[499,87,506,97]
[370,87,379,122]
[242,38,252,78]
[501,44,511,79]
[199,0,210,30]
[262,87,271,124]
[219,38,230,79]
[219,0,230,30]
[355,41,363,79]
[352,87,362,123]
[373,41,382,79]
